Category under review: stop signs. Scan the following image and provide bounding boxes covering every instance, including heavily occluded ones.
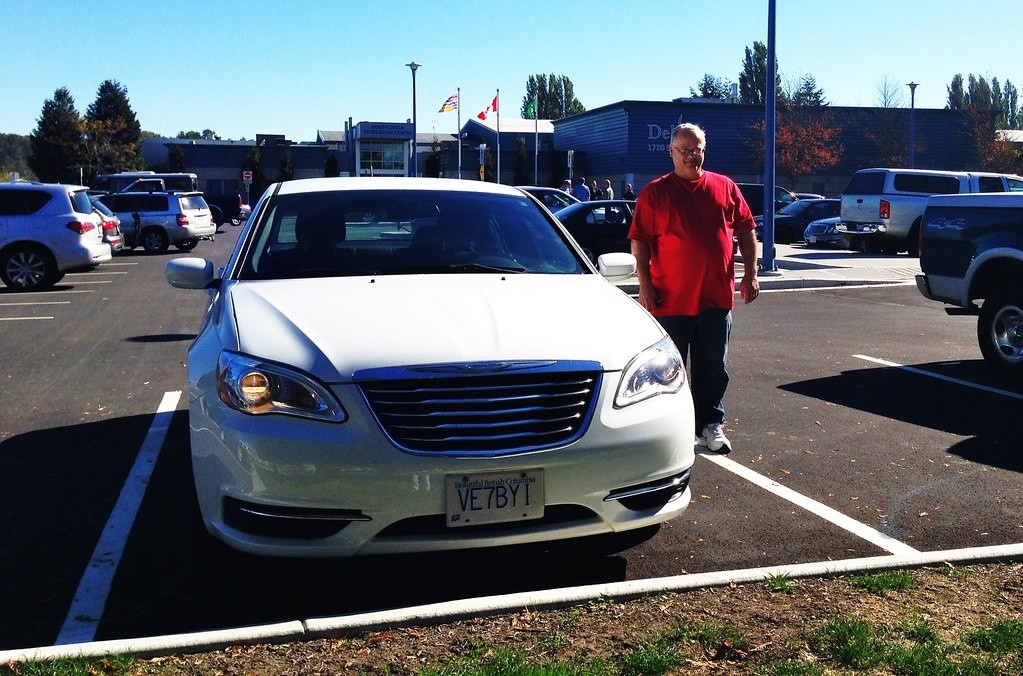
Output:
[244,172,251,179]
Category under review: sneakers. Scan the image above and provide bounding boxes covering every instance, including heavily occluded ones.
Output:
[699,423,732,453]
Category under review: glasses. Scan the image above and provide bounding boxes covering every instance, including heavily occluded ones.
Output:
[670,143,705,155]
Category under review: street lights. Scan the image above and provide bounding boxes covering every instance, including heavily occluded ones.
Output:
[406,62,423,176]
[906,82,919,168]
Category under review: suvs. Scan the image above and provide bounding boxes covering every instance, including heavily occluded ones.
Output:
[0,181,125,292]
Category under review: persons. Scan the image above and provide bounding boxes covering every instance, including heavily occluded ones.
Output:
[627,121,760,453]
[590,179,603,208]
[603,179,614,208]
[559,180,572,207]
[573,177,590,202]
[622,183,634,212]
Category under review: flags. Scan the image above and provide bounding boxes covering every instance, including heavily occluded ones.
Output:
[438,93,457,113]
[526,98,535,119]
[477,94,497,121]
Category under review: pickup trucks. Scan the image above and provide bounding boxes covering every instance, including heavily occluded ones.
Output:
[202,193,242,229]
[229,204,252,226]
[914,192,1023,384]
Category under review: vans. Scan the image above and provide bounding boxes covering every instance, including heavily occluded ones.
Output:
[735,183,825,215]
[837,168,1022,257]
[92,191,217,253]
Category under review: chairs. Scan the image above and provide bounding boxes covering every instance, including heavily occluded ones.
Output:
[283,204,501,269]
[605,210,625,223]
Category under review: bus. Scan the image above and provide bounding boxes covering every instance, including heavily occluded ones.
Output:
[98,171,198,192]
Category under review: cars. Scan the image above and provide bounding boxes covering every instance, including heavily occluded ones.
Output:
[166,177,695,562]
[804,216,871,254]
[749,199,842,242]
